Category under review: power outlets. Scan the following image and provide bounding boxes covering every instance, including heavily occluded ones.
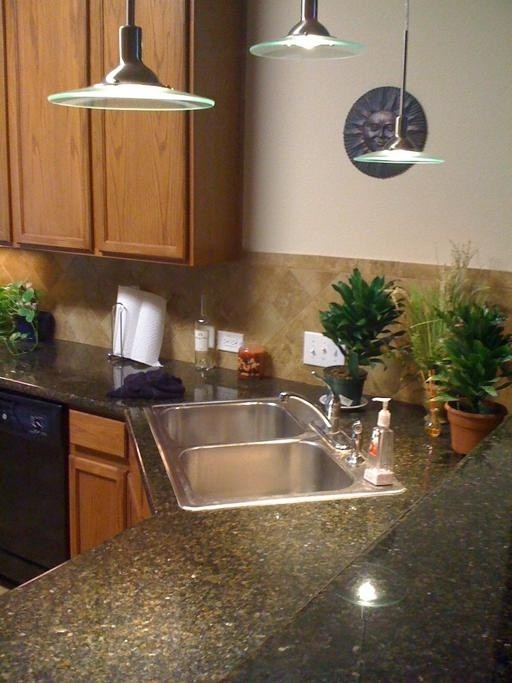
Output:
[301,331,347,370]
[216,330,245,354]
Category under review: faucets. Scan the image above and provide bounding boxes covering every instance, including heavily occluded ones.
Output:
[277,370,338,437]
[313,402,366,464]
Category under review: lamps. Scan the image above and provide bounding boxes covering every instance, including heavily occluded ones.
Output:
[45,1,216,113]
[250,1,445,166]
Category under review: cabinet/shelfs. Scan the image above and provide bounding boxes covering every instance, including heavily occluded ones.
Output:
[0,1,248,267]
[68,407,153,558]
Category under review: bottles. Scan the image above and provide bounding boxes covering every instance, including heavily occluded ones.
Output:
[239,346,265,380]
[194,293,209,371]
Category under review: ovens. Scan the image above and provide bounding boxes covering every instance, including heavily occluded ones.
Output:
[0,391,69,586]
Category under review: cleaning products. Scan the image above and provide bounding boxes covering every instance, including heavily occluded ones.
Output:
[362,397,395,486]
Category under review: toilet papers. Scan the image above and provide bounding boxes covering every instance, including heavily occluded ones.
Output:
[112,285,168,369]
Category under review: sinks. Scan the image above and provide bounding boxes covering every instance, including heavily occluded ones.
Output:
[156,439,408,514]
[144,394,325,445]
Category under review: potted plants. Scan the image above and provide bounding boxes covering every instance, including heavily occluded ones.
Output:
[318,267,411,411]
[403,243,484,436]
[438,303,512,454]
